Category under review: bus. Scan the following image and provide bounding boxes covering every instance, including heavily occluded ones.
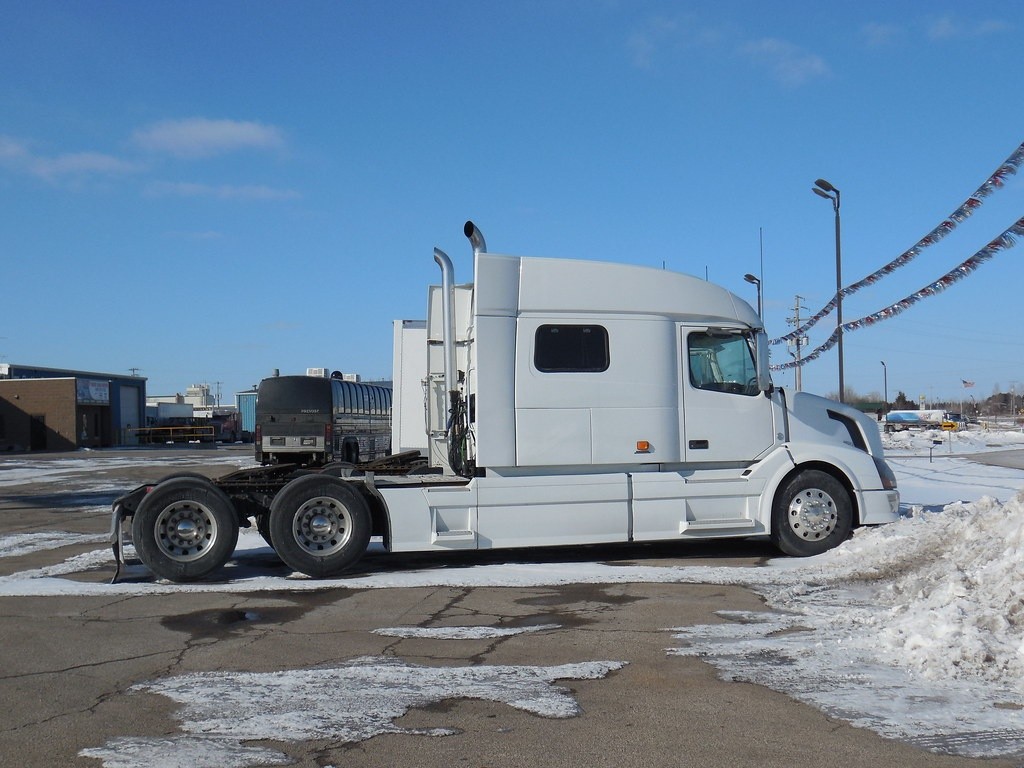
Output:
[160,416,212,443]
[253,371,393,464]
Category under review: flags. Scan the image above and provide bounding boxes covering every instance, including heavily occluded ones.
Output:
[962,380,976,388]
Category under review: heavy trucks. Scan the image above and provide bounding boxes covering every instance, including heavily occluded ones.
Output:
[145,402,194,436]
[884,410,961,433]
[205,393,257,444]
[108,220,901,583]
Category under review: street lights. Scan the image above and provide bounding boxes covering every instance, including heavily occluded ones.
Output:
[879,360,888,432]
[743,273,761,319]
[811,178,845,404]
